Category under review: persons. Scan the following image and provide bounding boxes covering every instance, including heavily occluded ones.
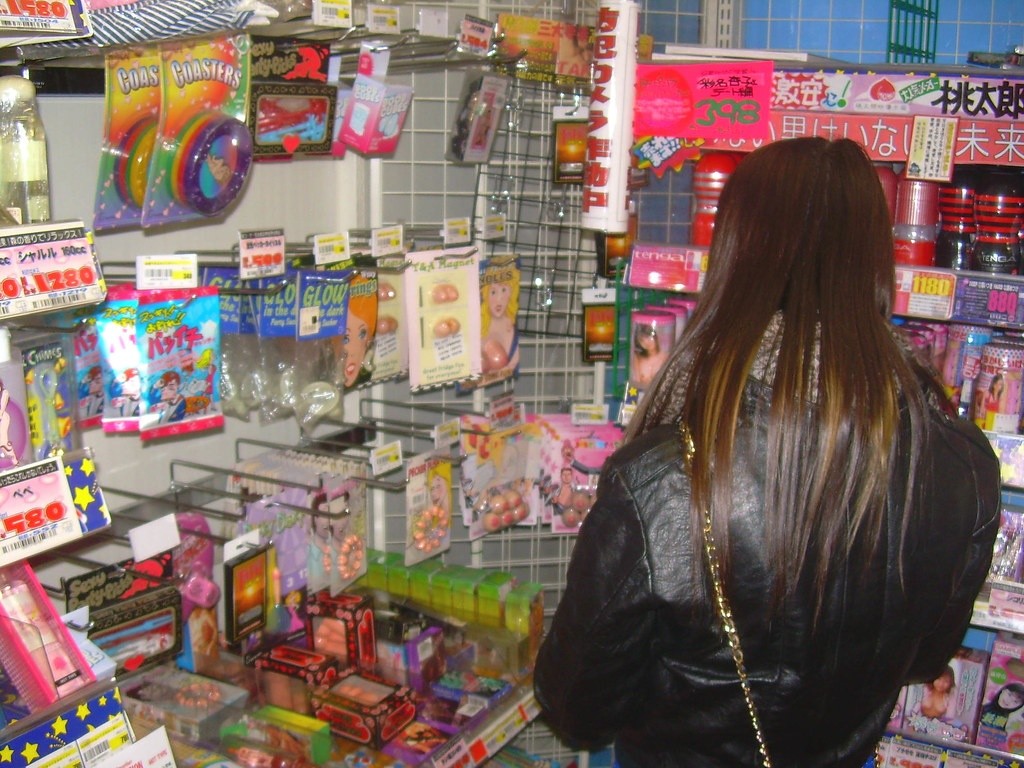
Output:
[631,324,669,386]
[532,136,1000,768]
[915,665,1024,731]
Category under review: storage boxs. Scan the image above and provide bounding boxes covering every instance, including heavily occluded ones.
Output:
[296,591,377,673]
[254,644,338,716]
[410,560,444,606]
[368,551,404,590]
[476,573,518,630]
[505,583,546,656]
[451,569,496,619]
[389,559,423,594]
[307,666,421,750]
[432,565,467,611]
[339,42,413,153]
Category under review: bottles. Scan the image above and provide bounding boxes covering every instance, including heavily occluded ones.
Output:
[691,150,1024,279]
